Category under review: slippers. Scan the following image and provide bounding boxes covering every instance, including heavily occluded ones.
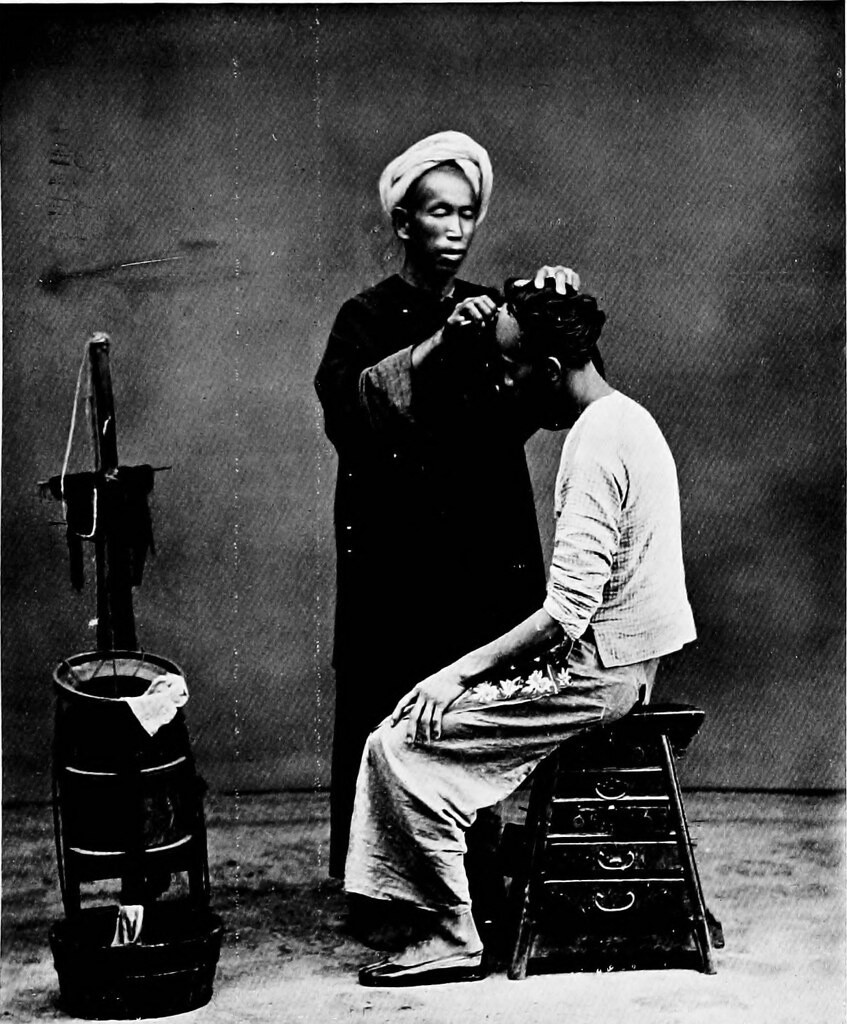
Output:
[360,948,482,981]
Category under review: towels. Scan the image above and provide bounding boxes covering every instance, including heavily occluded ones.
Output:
[119,670,190,738]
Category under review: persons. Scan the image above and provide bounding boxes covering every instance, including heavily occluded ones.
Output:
[355,278,698,988]
[312,127,578,944]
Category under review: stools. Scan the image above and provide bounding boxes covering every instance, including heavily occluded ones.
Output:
[498,700,716,979]
